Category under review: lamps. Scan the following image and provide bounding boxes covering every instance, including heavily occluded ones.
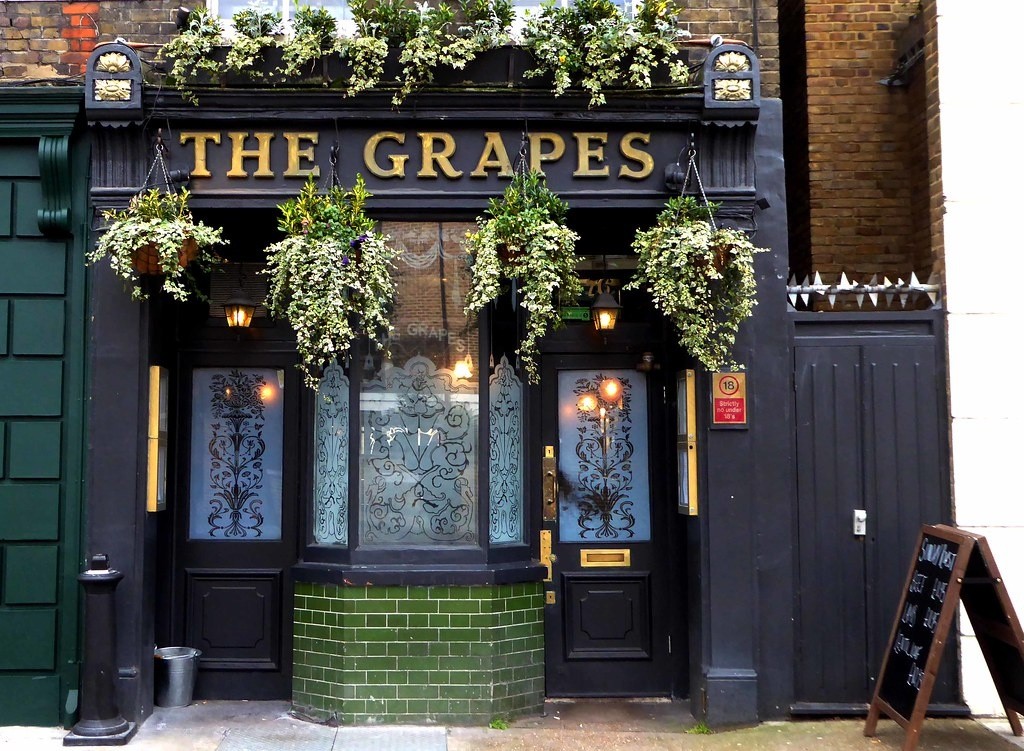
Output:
[220,263,259,343]
[592,255,621,345]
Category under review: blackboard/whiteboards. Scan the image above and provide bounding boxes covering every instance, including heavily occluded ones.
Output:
[862,521,1024,751]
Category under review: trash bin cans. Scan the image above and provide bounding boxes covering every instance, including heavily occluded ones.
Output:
[154,646,202,708]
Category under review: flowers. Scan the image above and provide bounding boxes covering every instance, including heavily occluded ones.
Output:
[253,171,404,389]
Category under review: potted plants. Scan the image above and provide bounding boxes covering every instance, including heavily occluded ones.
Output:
[464,172,586,387]
[85,191,230,302]
[623,195,772,370]
